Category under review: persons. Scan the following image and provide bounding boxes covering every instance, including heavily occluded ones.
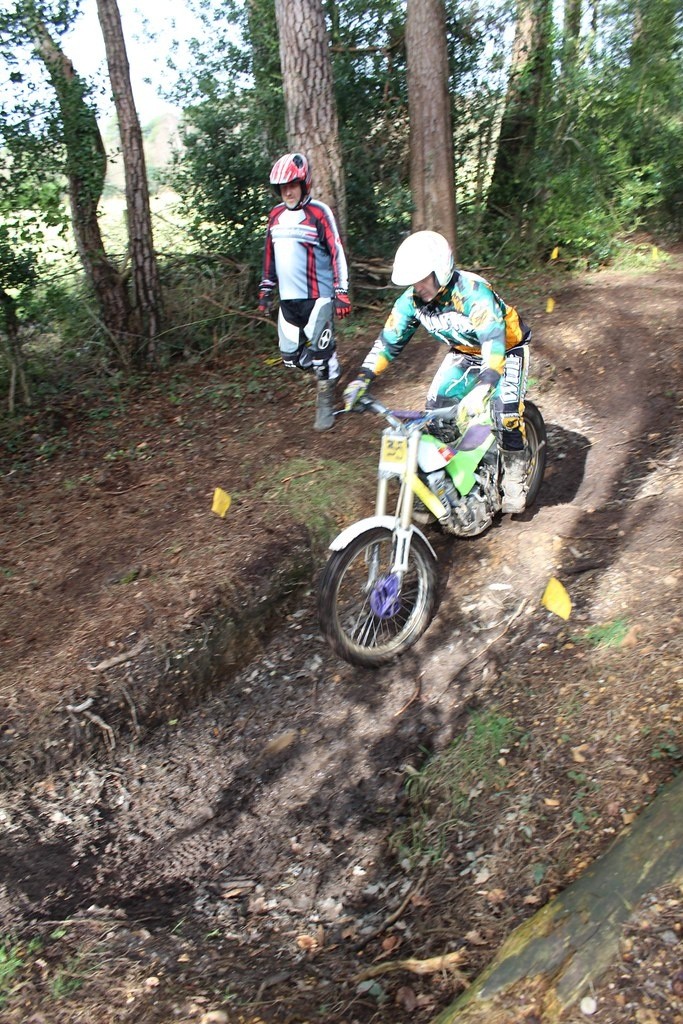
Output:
[257,152,354,432]
[342,228,534,524]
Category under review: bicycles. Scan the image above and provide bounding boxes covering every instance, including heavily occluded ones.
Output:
[318,375,553,674]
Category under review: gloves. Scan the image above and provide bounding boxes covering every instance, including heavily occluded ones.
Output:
[258,291,273,311]
[334,288,352,320]
[342,378,368,412]
[457,384,495,425]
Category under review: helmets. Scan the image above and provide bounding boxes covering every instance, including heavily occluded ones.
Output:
[270,153,312,210]
[392,230,454,286]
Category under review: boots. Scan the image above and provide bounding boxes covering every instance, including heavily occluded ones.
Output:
[497,439,530,513]
[313,380,336,430]
